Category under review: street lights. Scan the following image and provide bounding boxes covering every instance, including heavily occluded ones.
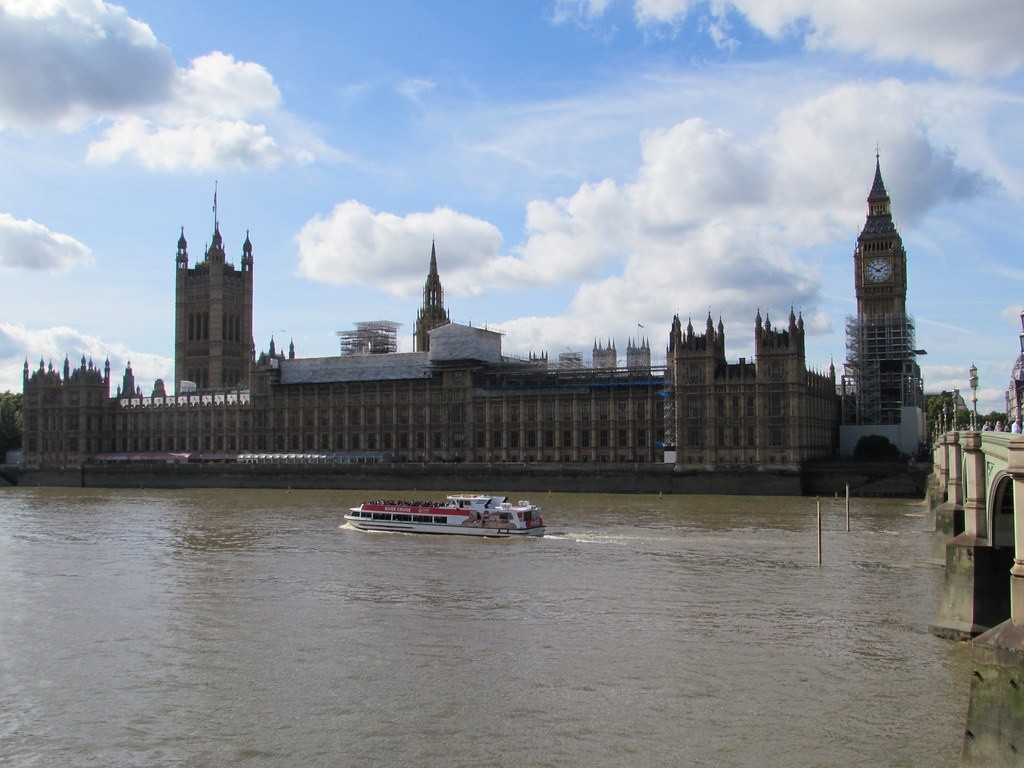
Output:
[1020,310,1024,330]
[969,362,978,431]
[1004,390,1010,432]
[935,421,938,440]
[928,428,930,436]
[942,401,948,433]
[938,413,942,436]
[931,432,935,444]
[1019,331,1024,353]
[951,387,959,431]
[969,412,973,431]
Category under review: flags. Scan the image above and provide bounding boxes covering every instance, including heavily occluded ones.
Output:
[638,324,644,328]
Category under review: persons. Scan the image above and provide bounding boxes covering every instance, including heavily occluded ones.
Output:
[958,419,1021,434]
[368,499,453,508]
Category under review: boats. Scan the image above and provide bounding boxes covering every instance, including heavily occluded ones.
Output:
[342,494,548,539]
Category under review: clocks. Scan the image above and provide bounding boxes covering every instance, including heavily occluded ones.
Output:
[865,257,892,283]
